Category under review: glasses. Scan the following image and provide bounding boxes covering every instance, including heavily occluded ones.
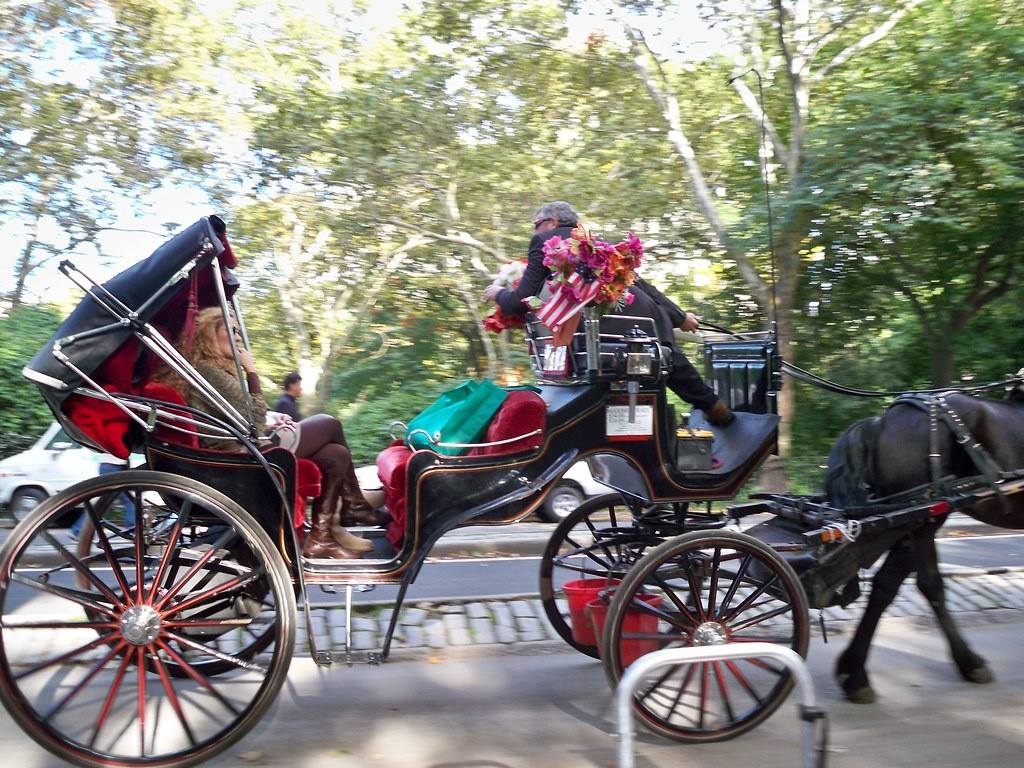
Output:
[534,218,561,230]
[232,326,239,335]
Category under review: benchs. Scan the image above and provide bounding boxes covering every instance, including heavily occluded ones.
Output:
[375,373,544,535]
[142,380,328,544]
[534,285,674,380]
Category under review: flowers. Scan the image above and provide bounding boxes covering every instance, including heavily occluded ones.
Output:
[543,221,646,305]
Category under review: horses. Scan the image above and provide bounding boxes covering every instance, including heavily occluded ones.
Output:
[824,385,1024,704]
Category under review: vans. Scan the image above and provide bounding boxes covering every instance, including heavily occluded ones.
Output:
[0,417,166,528]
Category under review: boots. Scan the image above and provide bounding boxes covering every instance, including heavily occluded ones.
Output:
[333,495,374,552]
[361,489,387,506]
[301,493,362,560]
[342,463,394,528]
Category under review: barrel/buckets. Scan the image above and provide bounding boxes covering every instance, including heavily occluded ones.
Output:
[586,592,663,668]
[561,579,625,647]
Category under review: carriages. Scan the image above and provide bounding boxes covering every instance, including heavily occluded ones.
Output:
[0,213,1024,768]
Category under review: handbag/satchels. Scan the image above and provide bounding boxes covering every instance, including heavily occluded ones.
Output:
[402,377,542,456]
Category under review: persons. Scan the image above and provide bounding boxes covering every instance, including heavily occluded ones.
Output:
[66,452,137,544]
[277,372,303,423]
[154,308,392,559]
[482,201,737,431]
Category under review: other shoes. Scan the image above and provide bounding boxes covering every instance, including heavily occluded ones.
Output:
[702,398,735,429]
[66,532,79,542]
